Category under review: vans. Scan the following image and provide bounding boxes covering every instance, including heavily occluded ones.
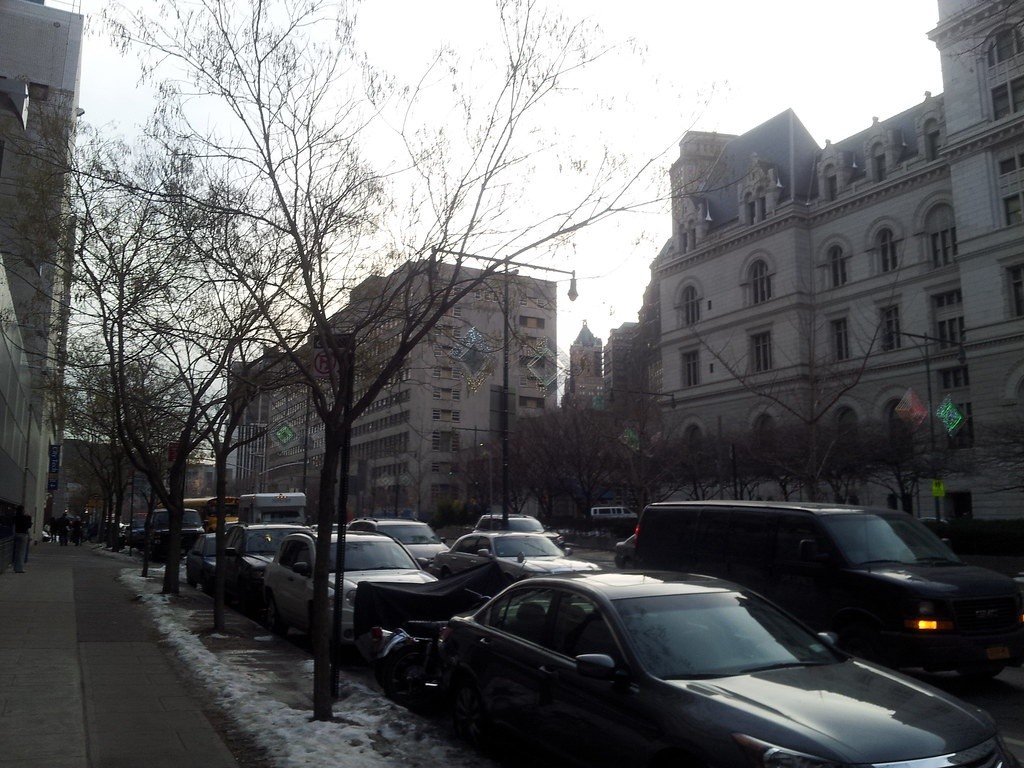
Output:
[591,506,638,519]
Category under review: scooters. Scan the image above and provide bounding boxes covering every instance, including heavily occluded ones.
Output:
[354,552,527,708]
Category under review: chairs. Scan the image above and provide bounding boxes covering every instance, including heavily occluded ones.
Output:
[507,601,545,645]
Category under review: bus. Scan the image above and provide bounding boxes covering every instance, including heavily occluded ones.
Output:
[238,493,306,523]
[183,497,239,532]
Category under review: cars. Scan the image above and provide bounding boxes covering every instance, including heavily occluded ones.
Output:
[439,569,1019,768]
[186,533,217,596]
[433,531,601,582]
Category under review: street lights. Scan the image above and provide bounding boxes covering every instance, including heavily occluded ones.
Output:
[429,248,579,507]
[451,422,500,497]
[374,448,417,517]
[881,330,967,536]
[607,387,677,507]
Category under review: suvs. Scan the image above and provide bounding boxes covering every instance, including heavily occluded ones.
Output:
[346,518,450,565]
[263,531,439,659]
[222,521,312,615]
[632,502,1016,681]
[475,514,564,551]
[145,507,203,554]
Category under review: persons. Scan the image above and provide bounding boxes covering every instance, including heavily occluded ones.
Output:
[12,504,32,574]
[49,510,87,547]
